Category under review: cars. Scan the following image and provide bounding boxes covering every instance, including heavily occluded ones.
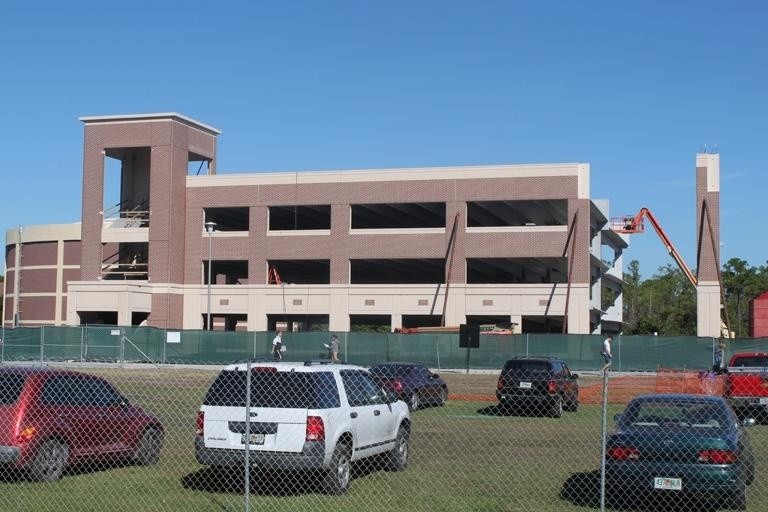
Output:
[598,394,754,509]
[371,362,448,410]
[0,363,164,482]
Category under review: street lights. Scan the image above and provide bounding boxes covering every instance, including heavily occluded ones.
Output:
[205,222,217,330]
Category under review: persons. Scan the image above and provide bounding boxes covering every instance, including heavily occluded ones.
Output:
[600,335,613,373]
[271,330,288,360]
[327,334,340,364]
[713,342,728,376]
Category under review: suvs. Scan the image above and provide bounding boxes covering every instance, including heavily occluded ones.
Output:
[194,356,411,494]
[494,354,578,417]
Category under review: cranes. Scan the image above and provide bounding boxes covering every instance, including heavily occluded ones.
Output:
[609,208,735,339]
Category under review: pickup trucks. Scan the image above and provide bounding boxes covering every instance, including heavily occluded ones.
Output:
[722,353,768,424]
